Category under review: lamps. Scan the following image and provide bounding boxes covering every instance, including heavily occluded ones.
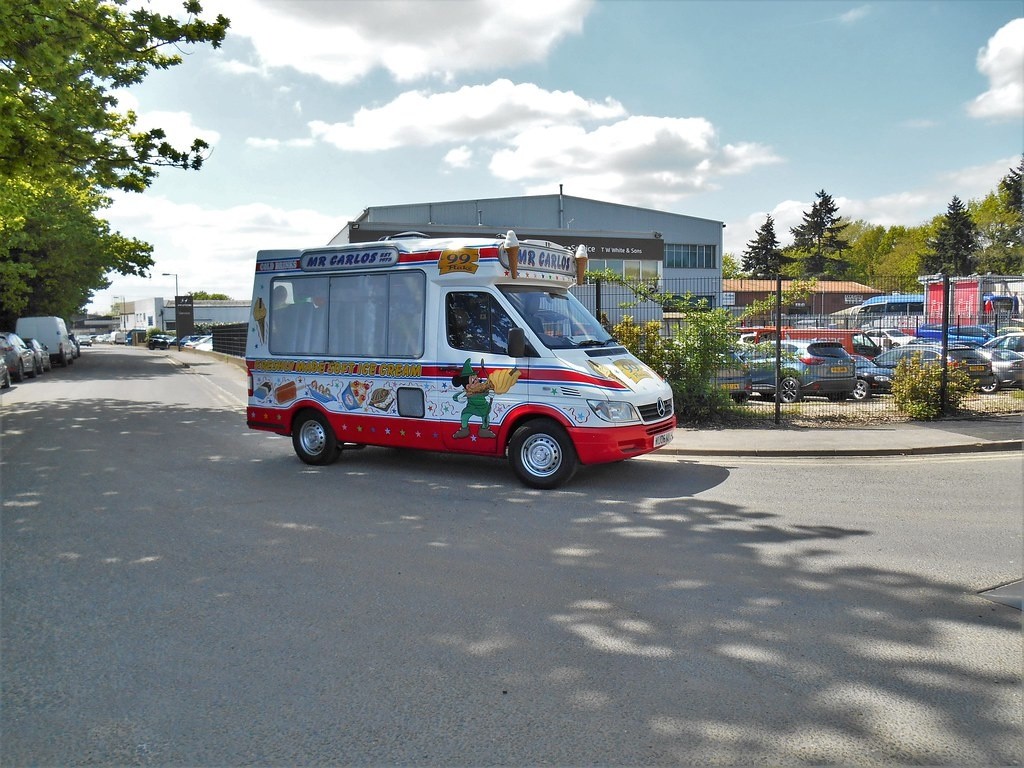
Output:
[722,224,726,229]
[352,223,360,229]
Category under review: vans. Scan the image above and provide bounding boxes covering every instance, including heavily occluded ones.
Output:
[108,332,127,344]
[733,326,883,360]
[16,317,75,367]
[125,329,146,345]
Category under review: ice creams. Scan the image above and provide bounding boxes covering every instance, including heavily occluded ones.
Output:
[504,230,520,279]
[253,297,266,343]
[585,359,636,392]
[575,244,587,285]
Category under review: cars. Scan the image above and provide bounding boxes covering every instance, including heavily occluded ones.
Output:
[0,352,11,389]
[663,346,753,403]
[169,336,213,352]
[974,347,1024,394]
[68,332,81,358]
[735,332,756,350]
[918,318,1024,355]
[794,314,831,327]
[90,333,111,343]
[853,328,917,347]
[848,354,898,402]
[77,335,92,347]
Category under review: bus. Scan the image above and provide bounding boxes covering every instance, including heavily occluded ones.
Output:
[849,294,1019,324]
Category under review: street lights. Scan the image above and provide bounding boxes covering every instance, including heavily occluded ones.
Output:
[114,296,126,328]
[757,273,772,323]
[163,273,178,296]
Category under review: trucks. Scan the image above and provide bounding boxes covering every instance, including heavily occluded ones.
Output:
[245,227,679,487]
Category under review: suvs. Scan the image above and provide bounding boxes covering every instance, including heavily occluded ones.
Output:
[23,337,52,374]
[741,340,858,404]
[869,343,994,392]
[146,335,173,350]
[0,332,37,383]
